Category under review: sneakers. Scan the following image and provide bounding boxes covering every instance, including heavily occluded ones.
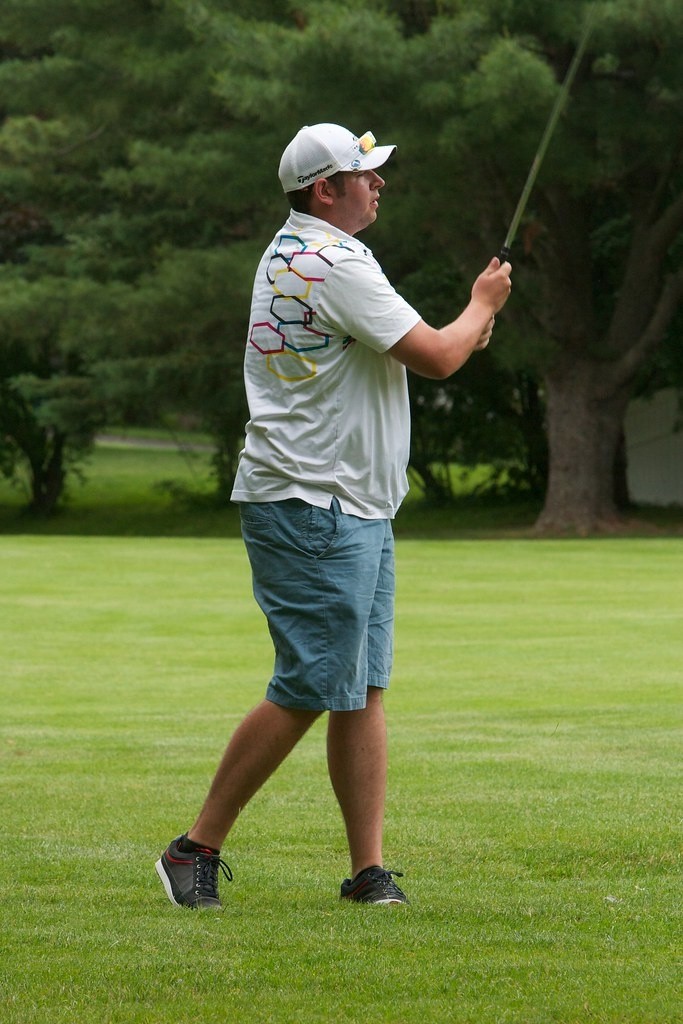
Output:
[341,864,407,904]
[155,832,232,908]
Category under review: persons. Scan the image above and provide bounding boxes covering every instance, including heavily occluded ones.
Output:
[156,122,512,907]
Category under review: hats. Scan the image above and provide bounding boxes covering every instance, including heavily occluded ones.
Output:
[278,123,397,192]
